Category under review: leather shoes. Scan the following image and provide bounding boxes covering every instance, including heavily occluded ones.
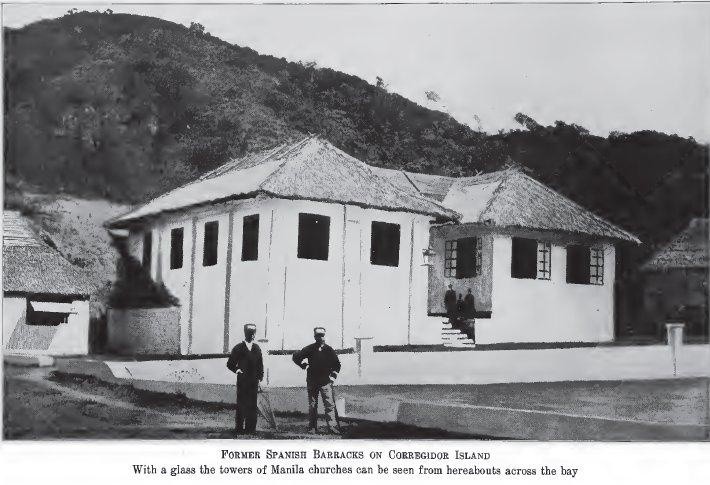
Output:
[308,428,315,434]
[328,426,340,434]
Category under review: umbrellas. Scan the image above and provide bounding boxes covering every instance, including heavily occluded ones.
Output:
[258,384,280,431]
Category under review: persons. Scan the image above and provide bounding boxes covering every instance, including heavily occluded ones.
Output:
[292,326,341,436]
[225,323,264,433]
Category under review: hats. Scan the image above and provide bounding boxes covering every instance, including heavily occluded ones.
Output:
[314,328,326,335]
[244,324,256,332]
